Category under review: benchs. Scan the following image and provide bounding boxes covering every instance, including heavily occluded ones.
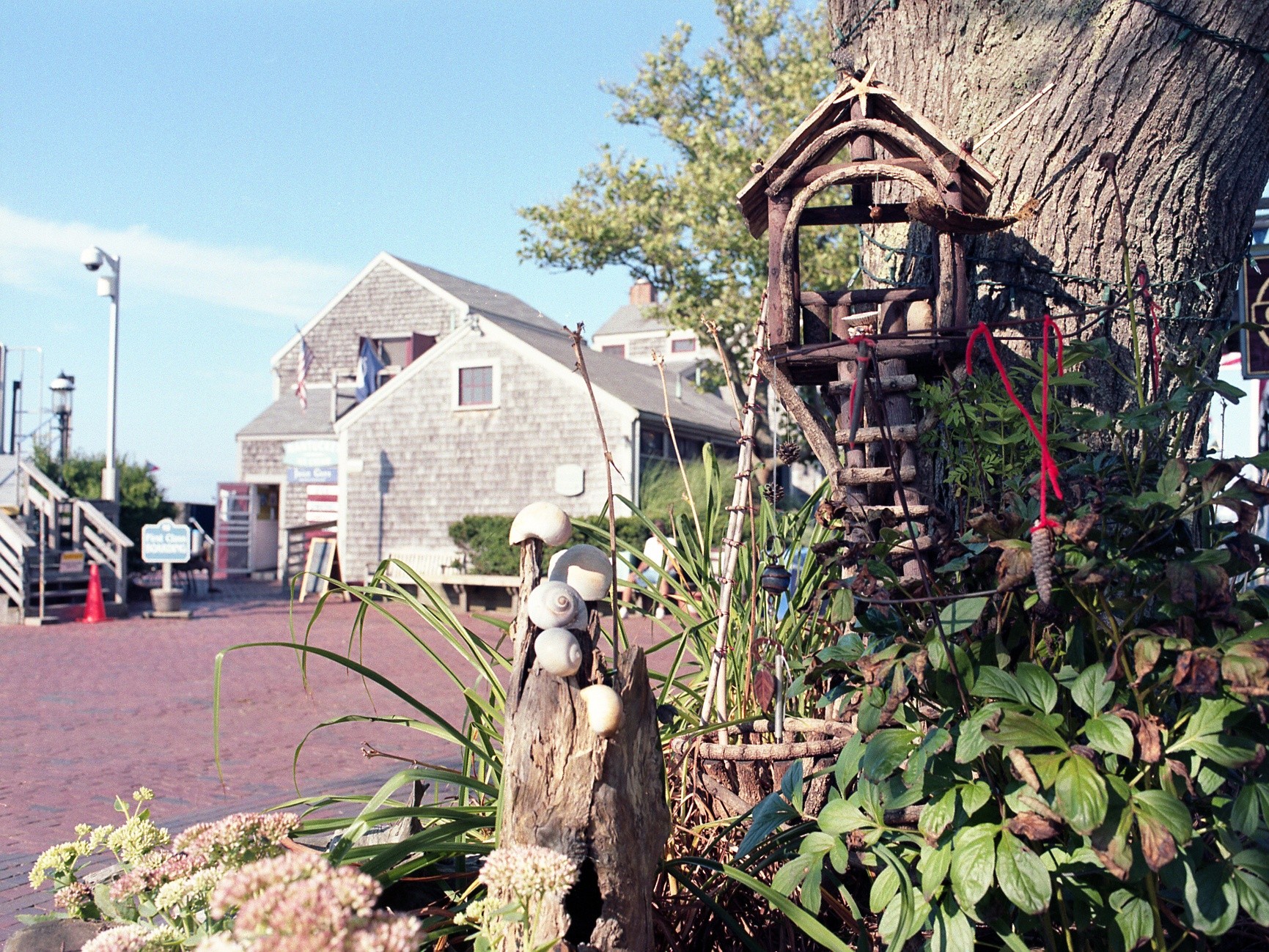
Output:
[361,544,721,620]
